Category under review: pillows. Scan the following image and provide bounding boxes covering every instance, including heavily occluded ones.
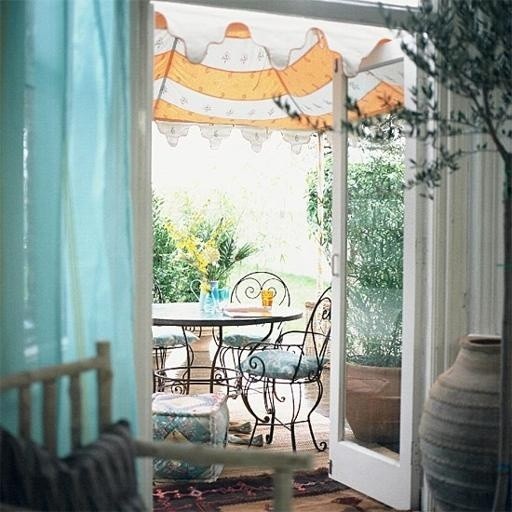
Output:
[0,420,148,512]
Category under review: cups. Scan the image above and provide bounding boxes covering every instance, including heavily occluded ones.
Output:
[259,289,274,309]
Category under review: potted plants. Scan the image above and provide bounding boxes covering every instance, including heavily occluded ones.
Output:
[306,156,406,444]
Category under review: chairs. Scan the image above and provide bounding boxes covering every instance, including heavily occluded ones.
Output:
[153,272,332,452]
[0,341,315,512]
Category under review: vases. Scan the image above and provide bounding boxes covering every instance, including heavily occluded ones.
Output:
[419,336,501,512]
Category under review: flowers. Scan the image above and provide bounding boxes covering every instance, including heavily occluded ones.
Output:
[164,188,258,293]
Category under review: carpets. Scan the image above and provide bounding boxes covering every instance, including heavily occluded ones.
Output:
[153,467,399,512]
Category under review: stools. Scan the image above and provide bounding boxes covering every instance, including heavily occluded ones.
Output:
[152,392,229,483]
